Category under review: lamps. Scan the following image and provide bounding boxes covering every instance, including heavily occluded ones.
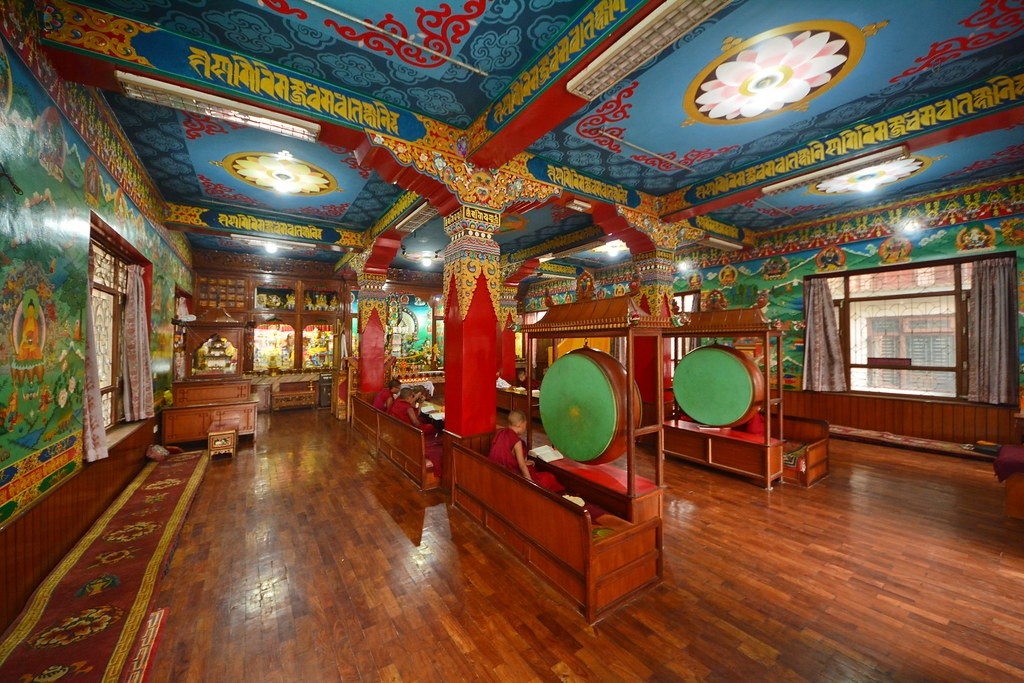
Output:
[698,236,743,253]
[762,145,911,198]
[536,272,576,281]
[114,70,321,146]
[566,199,592,212]
[229,233,317,251]
[395,199,439,234]
[566,0,734,103]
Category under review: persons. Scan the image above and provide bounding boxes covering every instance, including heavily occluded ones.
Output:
[486,408,565,492]
[730,411,764,436]
[373,379,401,411]
[517,370,538,387]
[388,387,437,434]
[394,385,427,418]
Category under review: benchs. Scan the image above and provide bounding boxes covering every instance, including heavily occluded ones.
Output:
[686,406,830,487]
[351,394,443,490]
[453,427,661,623]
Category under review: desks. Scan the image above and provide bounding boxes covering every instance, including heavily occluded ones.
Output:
[530,450,664,553]
[206,422,239,461]
[416,397,446,422]
[663,420,786,490]
[496,386,541,420]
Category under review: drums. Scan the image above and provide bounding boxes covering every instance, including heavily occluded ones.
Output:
[672,343,766,428]
[537,348,644,467]
